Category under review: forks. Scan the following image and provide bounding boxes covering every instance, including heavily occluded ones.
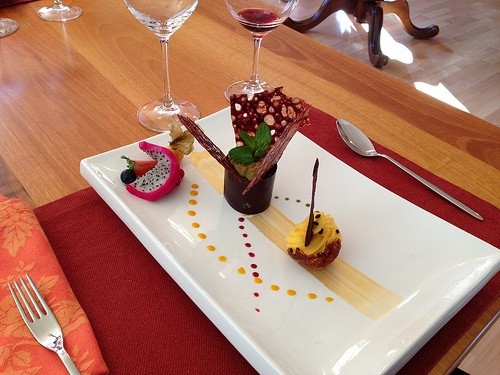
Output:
[7,273,83,375]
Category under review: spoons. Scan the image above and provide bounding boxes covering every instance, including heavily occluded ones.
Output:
[336,118,482,220]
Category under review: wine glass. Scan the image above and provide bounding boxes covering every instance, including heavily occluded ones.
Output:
[224,0,299,103]
[36,0,82,21]
[123,0,200,133]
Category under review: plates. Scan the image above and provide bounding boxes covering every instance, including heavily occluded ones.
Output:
[80,105,500,375]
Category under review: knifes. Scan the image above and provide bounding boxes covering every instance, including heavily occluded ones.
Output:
[303,158,319,247]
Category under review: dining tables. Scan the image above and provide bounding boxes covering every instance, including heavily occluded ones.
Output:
[0,0,500,375]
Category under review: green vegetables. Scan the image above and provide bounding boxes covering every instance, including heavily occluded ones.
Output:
[229,123,271,163]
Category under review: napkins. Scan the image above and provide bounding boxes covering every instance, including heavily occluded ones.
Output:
[0,193,111,375]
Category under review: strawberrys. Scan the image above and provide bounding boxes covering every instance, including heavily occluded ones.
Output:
[133,160,185,184]
